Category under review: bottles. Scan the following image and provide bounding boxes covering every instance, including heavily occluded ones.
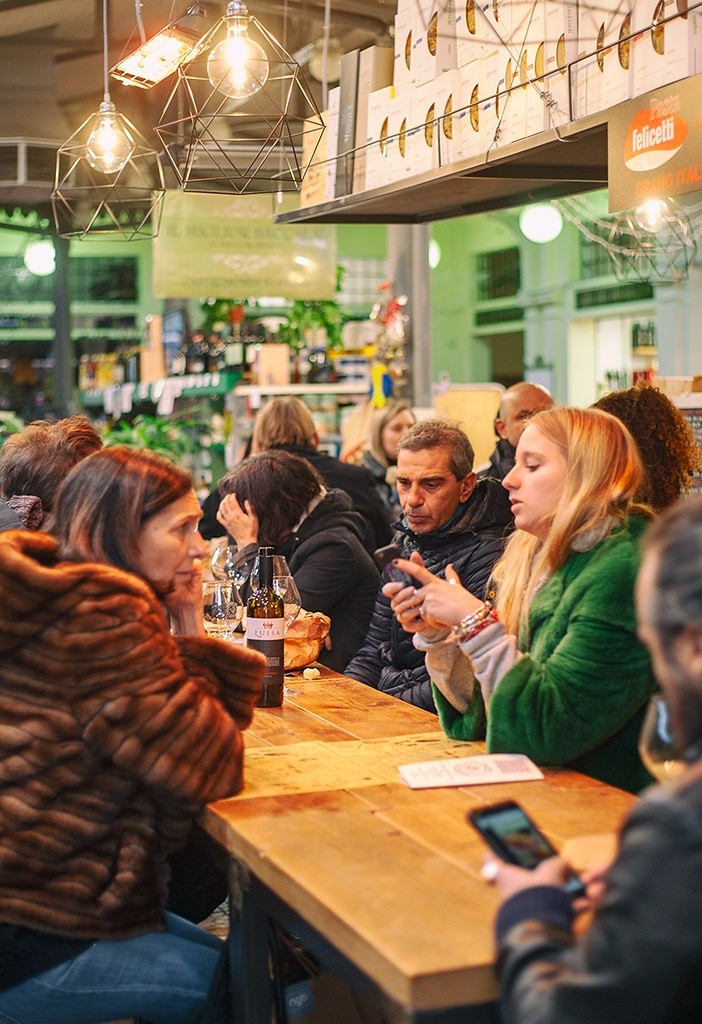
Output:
[172,325,269,374]
[79,346,140,388]
[248,546,284,708]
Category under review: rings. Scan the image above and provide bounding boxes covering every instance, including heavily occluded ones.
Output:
[419,607,424,615]
[484,861,498,881]
[222,511,225,517]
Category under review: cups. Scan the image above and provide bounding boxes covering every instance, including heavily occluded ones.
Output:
[202,581,244,640]
[250,555,292,597]
[210,545,250,591]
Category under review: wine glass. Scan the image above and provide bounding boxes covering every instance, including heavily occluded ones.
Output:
[272,576,304,697]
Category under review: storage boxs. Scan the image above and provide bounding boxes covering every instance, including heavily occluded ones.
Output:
[255,344,292,386]
[301,0,702,208]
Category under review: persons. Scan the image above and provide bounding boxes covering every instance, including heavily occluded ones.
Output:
[382,408,666,797]
[357,403,417,510]
[474,382,555,479]
[594,387,702,515]
[344,421,516,715]
[488,491,702,1024]
[0,444,268,1024]
[199,397,394,551]
[0,414,102,533]
[216,450,381,674]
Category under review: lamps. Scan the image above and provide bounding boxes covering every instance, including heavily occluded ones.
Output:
[109,0,211,88]
[606,197,696,286]
[154,0,327,196]
[50,0,170,239]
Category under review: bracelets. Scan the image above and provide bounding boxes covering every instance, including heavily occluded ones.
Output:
[456,601,499,643]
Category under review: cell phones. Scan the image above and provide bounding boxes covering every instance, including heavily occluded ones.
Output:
[467,798,588,901]
[373,544,426,621]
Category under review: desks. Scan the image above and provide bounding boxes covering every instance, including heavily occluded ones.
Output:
[198,659,644,1024]
